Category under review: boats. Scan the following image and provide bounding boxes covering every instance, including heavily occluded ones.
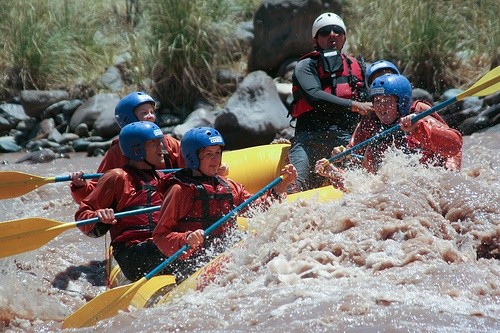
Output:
[103,139,370,314]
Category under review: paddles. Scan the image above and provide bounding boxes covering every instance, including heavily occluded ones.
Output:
[322,66,500,165]
[61,173,289,330]
[339,143,364,162]
[0,204,162,257]
[0,168,182,199]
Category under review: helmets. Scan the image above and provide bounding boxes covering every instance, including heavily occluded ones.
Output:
[181,125,225,169]
[114,91,156,128]
[312,12,346,39]
[119,120,164,162]
[365,60,400,88]
[368,72,413,115]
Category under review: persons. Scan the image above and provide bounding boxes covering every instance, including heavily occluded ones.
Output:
[284,12,375,194]
[66,91,298,286]
[315,59,463,187]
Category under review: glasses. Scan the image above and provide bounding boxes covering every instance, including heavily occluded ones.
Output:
[317,25,344,36]
